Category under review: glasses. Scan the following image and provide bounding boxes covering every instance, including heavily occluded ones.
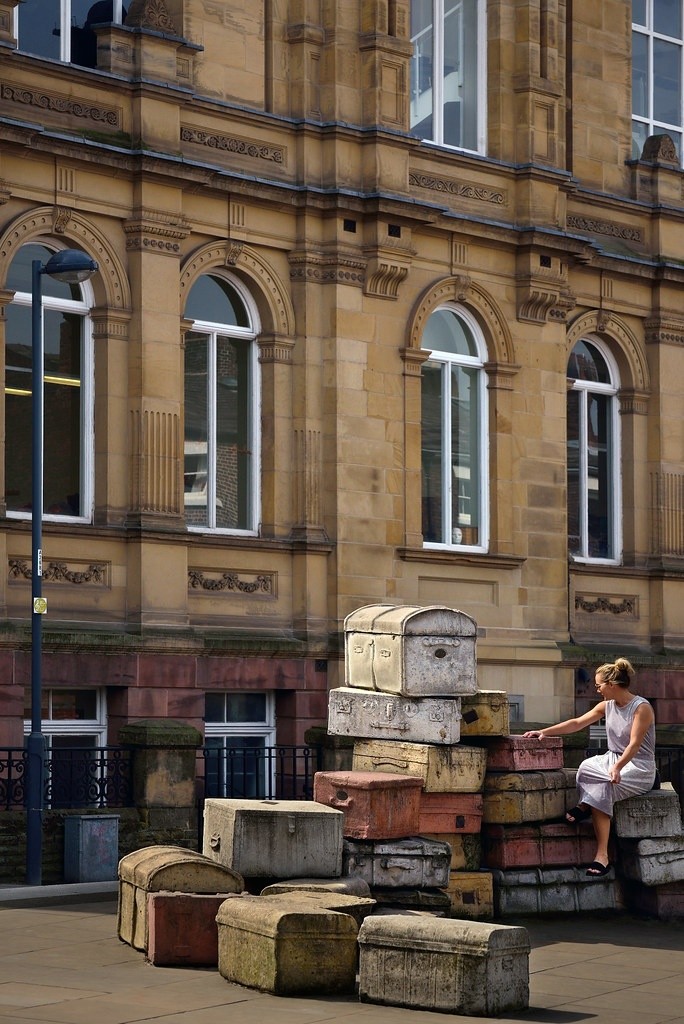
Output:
[595,682,609,688]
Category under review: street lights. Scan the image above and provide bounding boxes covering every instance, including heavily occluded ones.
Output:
[23,246,100,888]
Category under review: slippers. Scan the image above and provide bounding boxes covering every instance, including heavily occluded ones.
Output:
[586,862,612,876]
[565,807,592,825]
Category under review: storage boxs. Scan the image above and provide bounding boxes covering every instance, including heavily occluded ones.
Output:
[201,600,684,927]
[355,913,532,1018]
[113,844,244,968]
[215,894,358,995]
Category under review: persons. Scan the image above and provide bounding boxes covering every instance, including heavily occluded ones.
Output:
[522,658,656,877]
